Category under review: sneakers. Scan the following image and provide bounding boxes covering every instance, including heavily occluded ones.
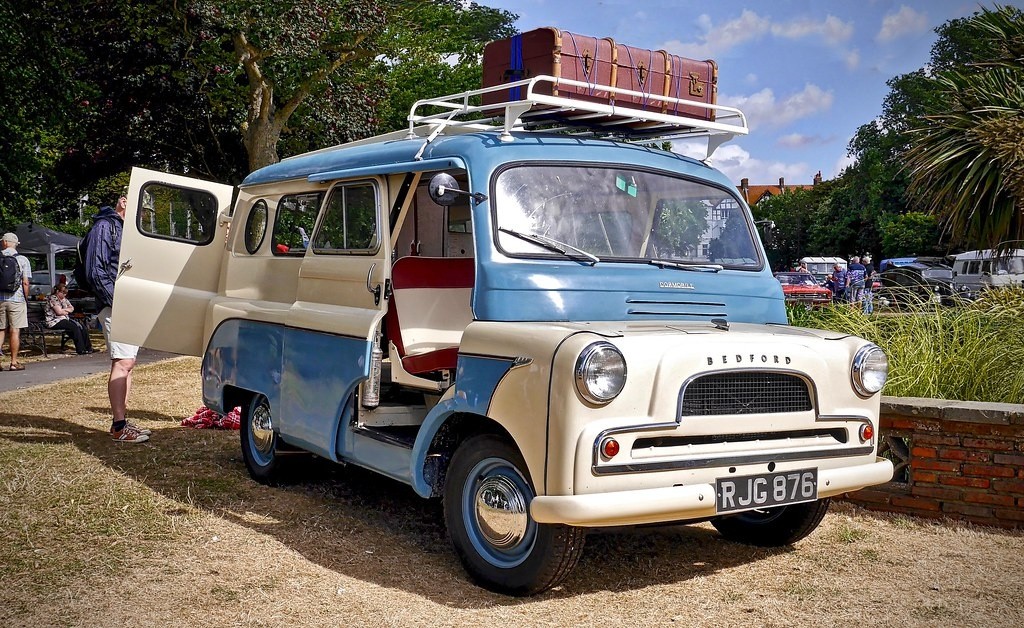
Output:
[110,424,152,435]
[112,425,149,443]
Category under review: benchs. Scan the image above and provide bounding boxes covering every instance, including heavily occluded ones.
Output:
[18,305,92,357]
[384,256,476,392]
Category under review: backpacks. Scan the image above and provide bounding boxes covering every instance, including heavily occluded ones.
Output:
[0,252,23,292]
[74,217,116,294]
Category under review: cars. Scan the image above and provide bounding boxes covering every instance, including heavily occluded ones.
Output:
[873,258,971,309]
[773,271,831,310]
[27,269,91,299]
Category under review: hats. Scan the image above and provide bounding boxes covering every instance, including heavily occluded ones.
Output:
[120,186,154,212]
[0,233,20,244]
[56,283,68,294]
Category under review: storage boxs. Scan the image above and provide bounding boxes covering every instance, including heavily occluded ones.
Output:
[482,27,718,125]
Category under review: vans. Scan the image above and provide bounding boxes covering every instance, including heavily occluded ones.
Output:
[952,247,1024,305]
[108,25,896,600]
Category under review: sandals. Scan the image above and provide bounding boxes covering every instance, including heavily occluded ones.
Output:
[10,361,25,370]
[0,366,3,371]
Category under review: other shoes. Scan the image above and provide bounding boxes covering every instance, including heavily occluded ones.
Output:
[79,349,92,354]
[89,348,100,352]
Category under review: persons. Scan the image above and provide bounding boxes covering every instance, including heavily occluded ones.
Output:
[44,283,100,354]
[794,256,875,314]
[80,186,154,443]
[0,232,32,370]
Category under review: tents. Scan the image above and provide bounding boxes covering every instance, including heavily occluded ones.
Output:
[0,221,84,288]
